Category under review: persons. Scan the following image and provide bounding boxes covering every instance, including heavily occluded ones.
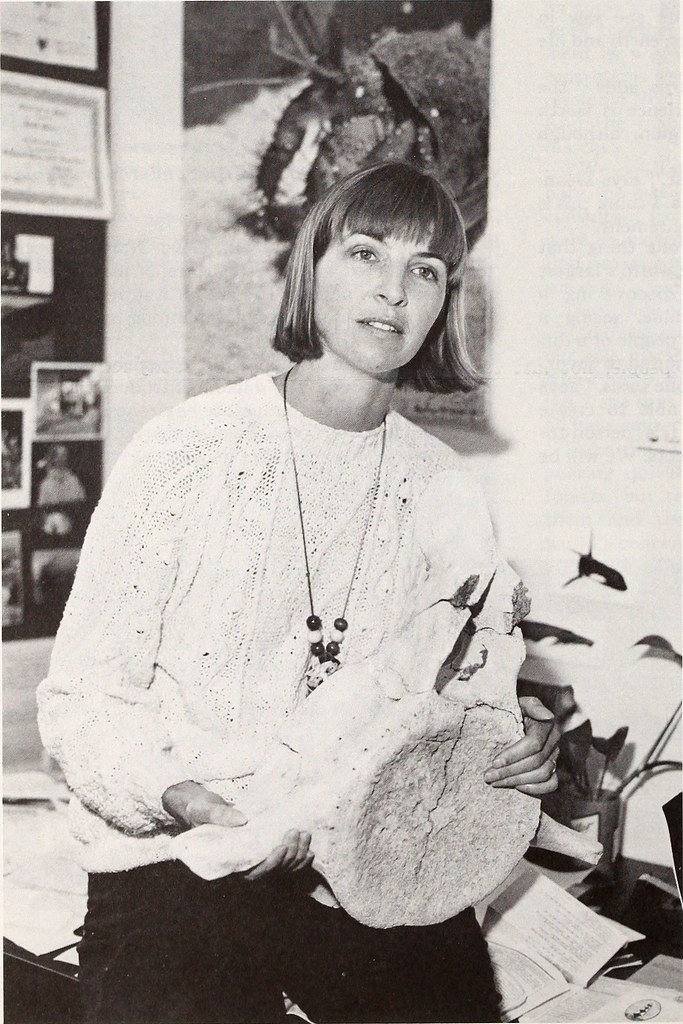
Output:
[33,158,563,1022]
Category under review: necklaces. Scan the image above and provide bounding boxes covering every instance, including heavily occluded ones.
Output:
[281,364,387,698]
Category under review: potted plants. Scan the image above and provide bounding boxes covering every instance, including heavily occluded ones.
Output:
[516,538,683,882]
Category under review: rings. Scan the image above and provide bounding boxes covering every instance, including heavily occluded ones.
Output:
[551,759,558,774]
